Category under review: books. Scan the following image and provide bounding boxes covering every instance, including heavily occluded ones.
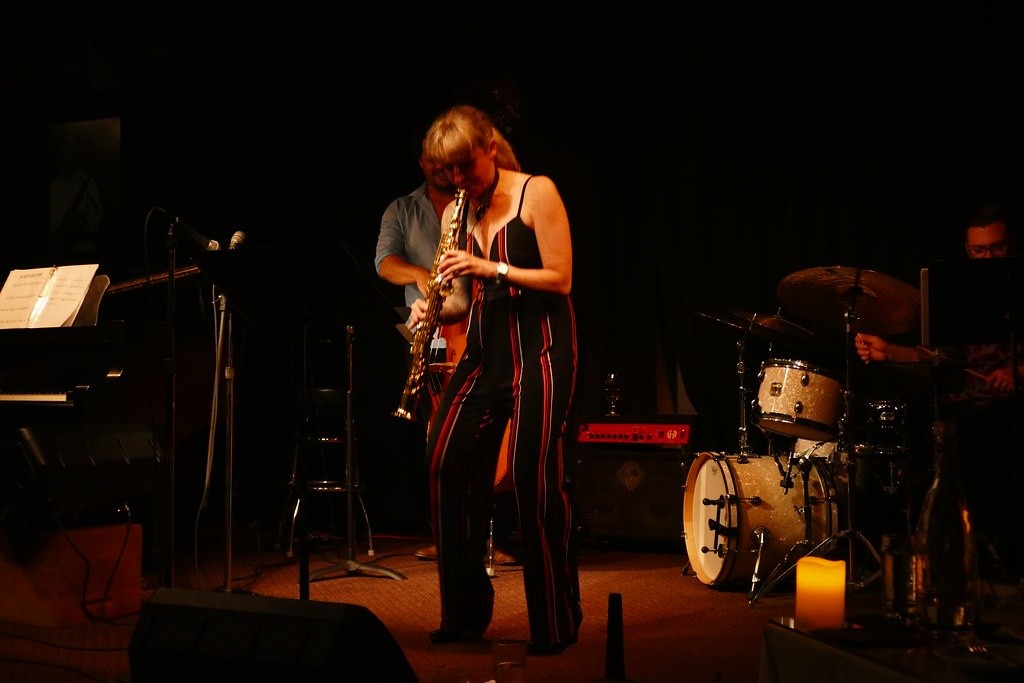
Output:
[0,261,104,330]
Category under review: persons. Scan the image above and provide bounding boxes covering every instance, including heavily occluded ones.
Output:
[408,105,583,656]
[855,202,1024,396]
[374,137,521,565]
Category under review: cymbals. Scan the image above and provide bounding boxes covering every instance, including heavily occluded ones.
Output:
[697,310,768,338]
[776,265,920,335]
[724,308,815,335]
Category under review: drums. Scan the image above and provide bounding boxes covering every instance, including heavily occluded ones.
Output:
[849,397,913,458]
[681,451,840,588]
[749,356,852,442]
[792,437,874,496]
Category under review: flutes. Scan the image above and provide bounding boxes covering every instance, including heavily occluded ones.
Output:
[390,187,469,423]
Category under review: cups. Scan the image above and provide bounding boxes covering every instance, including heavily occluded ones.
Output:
[491,639,528,683]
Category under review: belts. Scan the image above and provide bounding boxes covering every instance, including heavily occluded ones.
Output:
[440,305,470,325]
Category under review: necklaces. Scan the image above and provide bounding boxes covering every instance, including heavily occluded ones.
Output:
[467,169,499,240]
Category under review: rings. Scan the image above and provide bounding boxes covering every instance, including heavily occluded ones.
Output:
[1001,381,1009,390]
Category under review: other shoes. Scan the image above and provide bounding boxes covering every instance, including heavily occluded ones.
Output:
[414,546,438,560]
[430,628,481,644]
[527,612,583,656]
[494,551,518,564]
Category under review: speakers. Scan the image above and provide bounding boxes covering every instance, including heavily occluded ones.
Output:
[571,446,698,553]
[127,587,420,683]
[0,420,167,533]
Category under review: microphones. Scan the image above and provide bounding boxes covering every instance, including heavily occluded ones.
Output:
[161,211,222,253]
[229,231,248,252]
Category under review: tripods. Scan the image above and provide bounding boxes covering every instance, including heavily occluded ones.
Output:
[189,242,410,584]
[745,315,911,612]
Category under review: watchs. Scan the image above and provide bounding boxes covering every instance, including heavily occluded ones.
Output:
[496,262,508,284]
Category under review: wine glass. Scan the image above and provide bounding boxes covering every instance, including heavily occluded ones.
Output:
[601,371,624,417]
